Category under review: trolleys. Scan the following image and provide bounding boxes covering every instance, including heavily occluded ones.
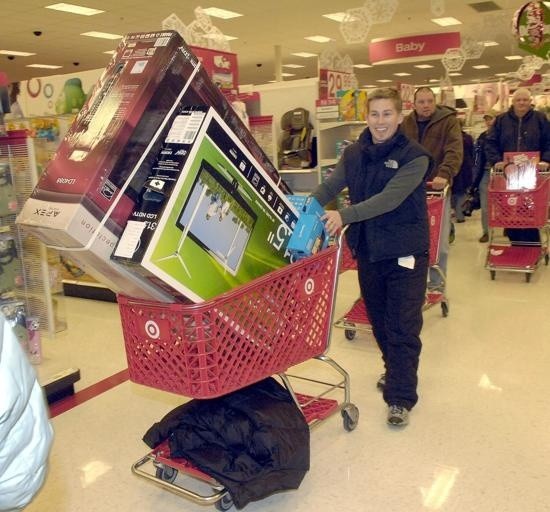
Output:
[483,159,550,284]
[115,215,360,512]
[332,176,454,341]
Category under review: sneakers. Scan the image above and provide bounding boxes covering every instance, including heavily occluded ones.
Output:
[480,233,489,243]
[386,404,408,426]
[449,222,455,243]
[378,374,386,388]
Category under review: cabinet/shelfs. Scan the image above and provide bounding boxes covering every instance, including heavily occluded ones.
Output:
[321,118,379,170]
[1,128,69,337]
[0,133,82,405]
[235,78,323,193]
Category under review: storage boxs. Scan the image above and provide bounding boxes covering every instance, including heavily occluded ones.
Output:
[502,151,541,191]
[335,88,368,122]
[21,32,292,310]
[109,103,299,307]
[276,191,328,259]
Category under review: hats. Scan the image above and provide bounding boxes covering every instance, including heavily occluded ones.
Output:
[483,109,497,118]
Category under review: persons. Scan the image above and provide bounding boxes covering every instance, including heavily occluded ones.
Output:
[304,85,435,427]
[0,310,54,511]
[401,86,463,294]
[450,90,550,266]
